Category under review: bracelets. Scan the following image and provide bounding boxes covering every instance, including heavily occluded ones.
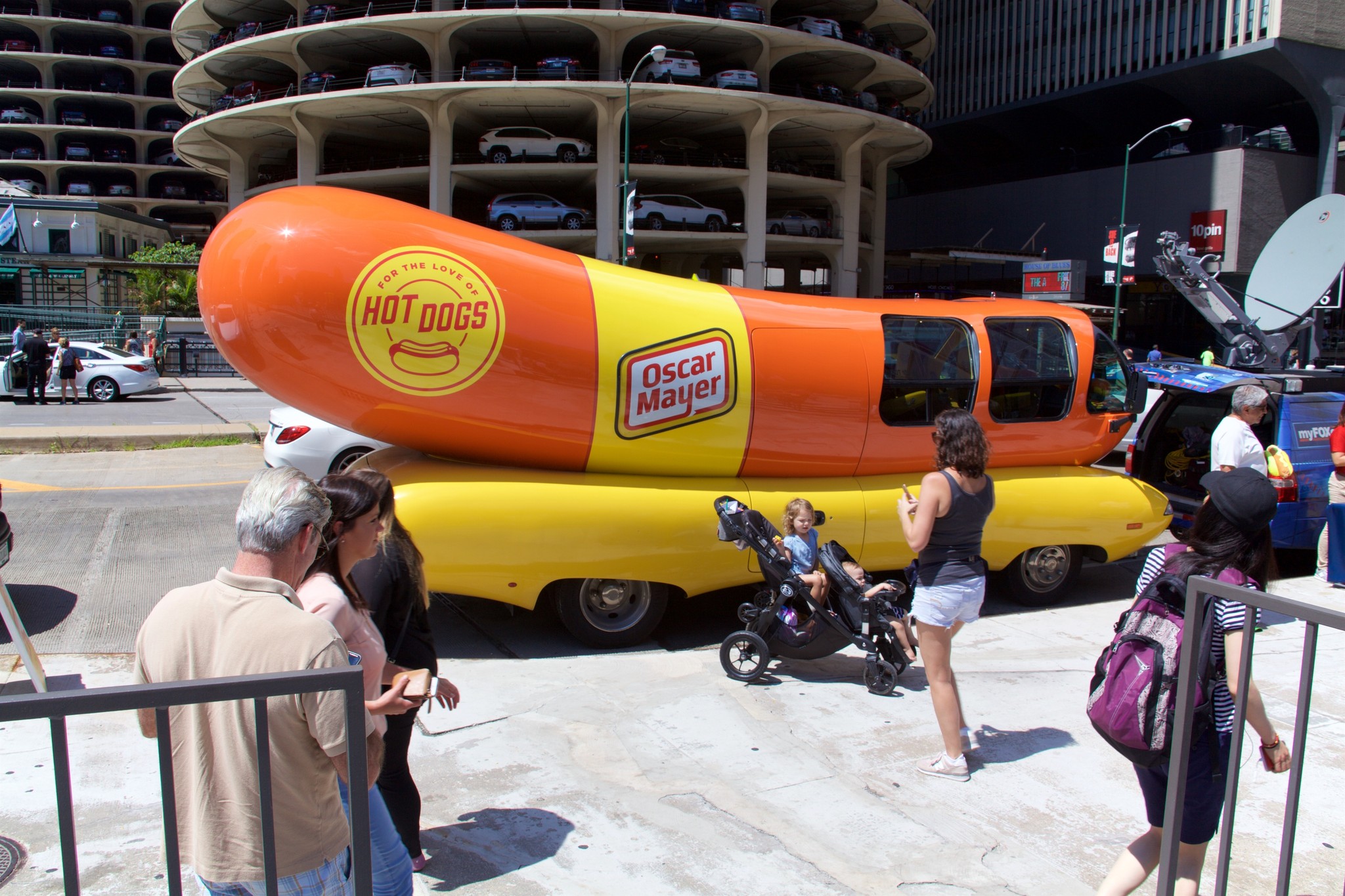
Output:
[1261,732,1279,748]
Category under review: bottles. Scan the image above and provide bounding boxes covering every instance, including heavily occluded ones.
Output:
[776,605,788,621]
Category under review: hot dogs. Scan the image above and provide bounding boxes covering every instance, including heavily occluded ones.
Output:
[1265,444,1293,478]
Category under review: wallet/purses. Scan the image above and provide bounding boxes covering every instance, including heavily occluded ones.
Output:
[389,669,434,704]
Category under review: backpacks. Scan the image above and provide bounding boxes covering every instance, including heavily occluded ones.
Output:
[1085,544,1261,765]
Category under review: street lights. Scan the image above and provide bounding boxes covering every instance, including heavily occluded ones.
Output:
[1111,118,1194,343]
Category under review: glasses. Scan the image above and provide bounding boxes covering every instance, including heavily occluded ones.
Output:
[54,333,60,334]
[302,521,330,561]
[1249,404,1268,411]
[931,430,942,445]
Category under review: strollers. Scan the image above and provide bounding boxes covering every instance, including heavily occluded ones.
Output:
[709,493,914,696]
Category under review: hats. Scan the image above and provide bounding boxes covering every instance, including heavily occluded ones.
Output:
[34,328,42,334]
[1199,467,1278,531]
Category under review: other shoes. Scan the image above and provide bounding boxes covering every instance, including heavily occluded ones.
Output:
[39,397,48,405]
[410,852,426,871]
[60,401,66,405]
[72,400,80,405]
[27,399,35,405]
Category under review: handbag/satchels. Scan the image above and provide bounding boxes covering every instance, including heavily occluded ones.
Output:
[155,344,165,357]
[71,347,84,373]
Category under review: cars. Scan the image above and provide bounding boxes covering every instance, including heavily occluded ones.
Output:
[537,56,587,81]
[773,14,923,128]
[634,134,739,168]
[205,20,285,50]
[702,69,758,91]
[263,404,395,490]
[668,0,766,26]
[464,52,518,81]
[300,70,354,95]
[764,151,833,239]
[302,4,362,28]
[362,60,430,88]
[212,79,291,114]
[1,9,226,201]
[634,47,702,86]
[0,338,160,403]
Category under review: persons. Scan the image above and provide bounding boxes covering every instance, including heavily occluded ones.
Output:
[125,331,145,356]
[897,408,996,783]
[1094,467,1292,896]
[13,319,26,352]
[49,327,60,343]
[295,468,460,896]
[1123,349,1136,364]
[772,498,838,619]
[56,337,80,405]
[1289,349,1299,369]
[1147,344,1162,363]
[146,330,161,374]
[842,561,920,660]
[134,465,385,896]
[22,328,50,405]
[1200,346,1214,366]
[1210,385,1268,478]
[1314,402,1345,583]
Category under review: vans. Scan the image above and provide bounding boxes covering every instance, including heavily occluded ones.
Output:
[1088,359,1344,561]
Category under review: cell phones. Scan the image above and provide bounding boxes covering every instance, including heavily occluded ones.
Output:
[348,650,362,666]
[902,484,912,500]
[1259,741,1285,772]
[409,676,439,702]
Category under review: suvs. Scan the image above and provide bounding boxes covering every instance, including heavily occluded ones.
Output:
[473,126,594,164]
[620,193,728,232]
[485,190,593,231]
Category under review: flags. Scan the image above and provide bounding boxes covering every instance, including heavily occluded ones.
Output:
[0,202,19,246]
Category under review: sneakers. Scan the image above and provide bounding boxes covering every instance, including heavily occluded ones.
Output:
[916,750,970,782]
[1313,568,1334,584]
[943,727,972,756]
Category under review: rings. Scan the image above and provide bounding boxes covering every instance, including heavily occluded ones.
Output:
[897,506,899,508]
